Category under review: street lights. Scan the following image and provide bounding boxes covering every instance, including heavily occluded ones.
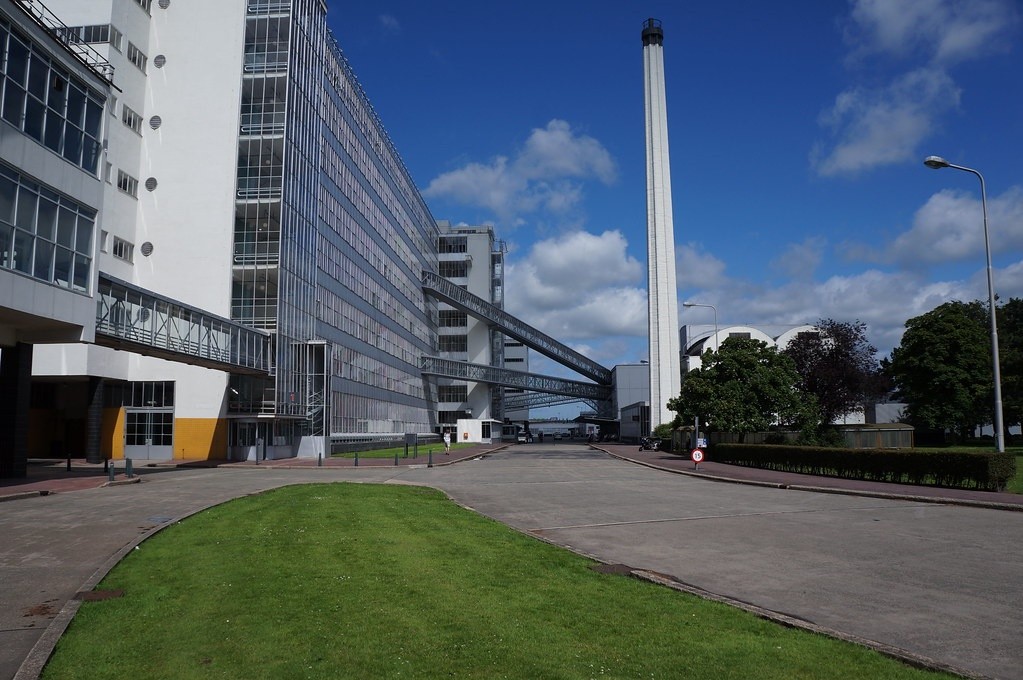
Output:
[682,301,720,365]
[923,154,1006,454]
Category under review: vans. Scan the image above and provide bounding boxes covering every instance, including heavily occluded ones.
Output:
[517,431,527,443]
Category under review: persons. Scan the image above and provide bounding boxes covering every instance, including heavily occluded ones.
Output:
[443,429,451,455]
[525,431,544,443]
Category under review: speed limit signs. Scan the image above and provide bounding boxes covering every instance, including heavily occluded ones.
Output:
[691,449,704,463]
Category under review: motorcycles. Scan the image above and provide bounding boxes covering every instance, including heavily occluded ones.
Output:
[638,435,663,451]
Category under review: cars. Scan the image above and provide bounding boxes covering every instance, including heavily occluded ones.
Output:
[554,433,562,441]
